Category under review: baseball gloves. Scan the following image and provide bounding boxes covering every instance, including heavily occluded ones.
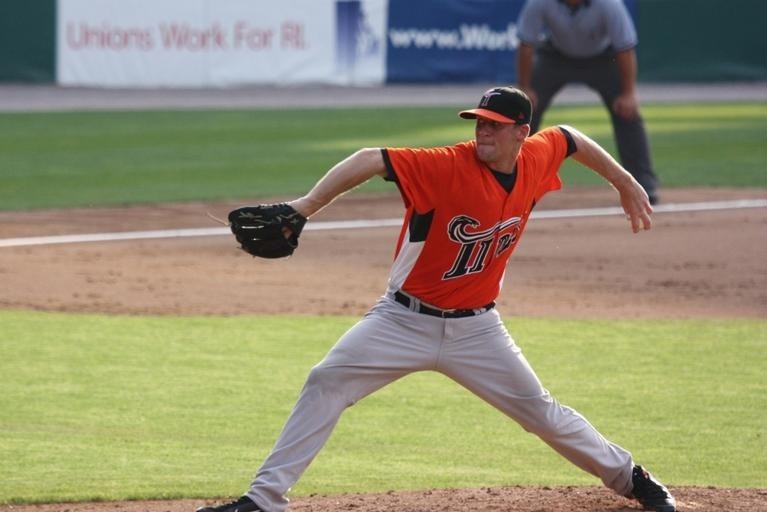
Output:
[228,203,310,258]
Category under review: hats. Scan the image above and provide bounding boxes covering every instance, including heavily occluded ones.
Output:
[457,85,535,125]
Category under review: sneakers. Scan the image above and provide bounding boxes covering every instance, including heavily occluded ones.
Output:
[632,463,678,510]
[196,495,262,512]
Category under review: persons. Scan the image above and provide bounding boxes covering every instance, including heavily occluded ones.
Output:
[514,1,658,205]
[192,84,678,512]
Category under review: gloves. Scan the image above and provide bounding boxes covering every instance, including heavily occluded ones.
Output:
[226,201,308,264]
